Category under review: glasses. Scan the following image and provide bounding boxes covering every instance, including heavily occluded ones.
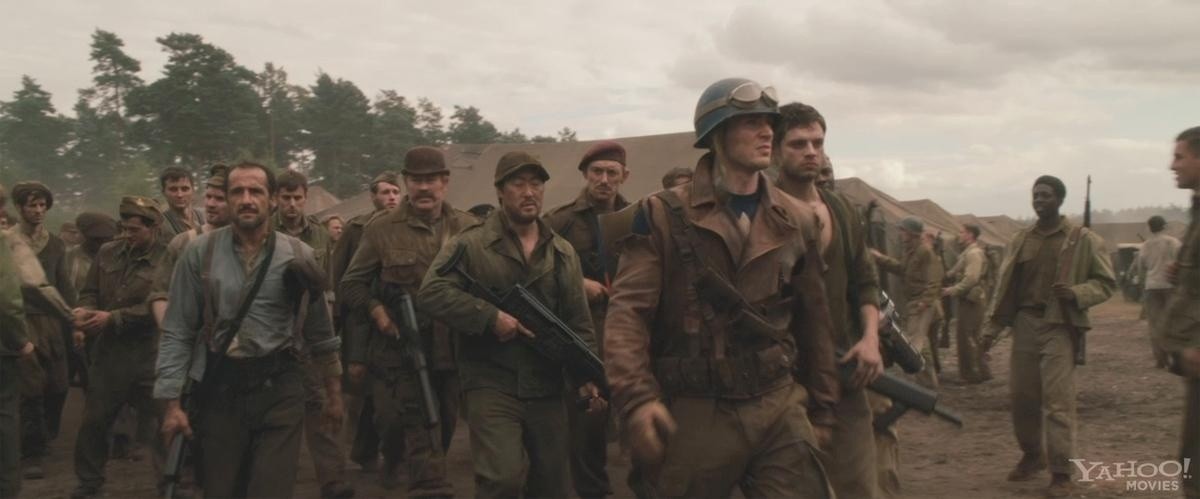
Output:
[727,82,781,108]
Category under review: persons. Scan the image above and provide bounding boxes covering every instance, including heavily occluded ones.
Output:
[0,77,1197,499]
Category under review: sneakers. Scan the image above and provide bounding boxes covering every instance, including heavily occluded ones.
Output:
[1049,476,1072,497]
[22,456,44,480]
[1008,452,1050,481]
[115,433,147,460]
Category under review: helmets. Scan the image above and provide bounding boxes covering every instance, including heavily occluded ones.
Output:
[898,216,923,235]
[692,78,785,149]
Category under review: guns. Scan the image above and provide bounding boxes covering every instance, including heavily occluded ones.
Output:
[879,289,926,373]
[1074,176,1092,364]
[835,348,964,427]
[159,380,195,499]
[393,290,442,455]
[435,241,610,413]
[933,231,953,349]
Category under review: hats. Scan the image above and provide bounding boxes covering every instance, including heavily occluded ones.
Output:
[201,163,232,189]
[12,182,52,211]
[372,170,404,193]
[494,152,550,185]
[75,212,119,240]
[400,147,451,176]
[579,141,626,170]
[119,197,166,226]
